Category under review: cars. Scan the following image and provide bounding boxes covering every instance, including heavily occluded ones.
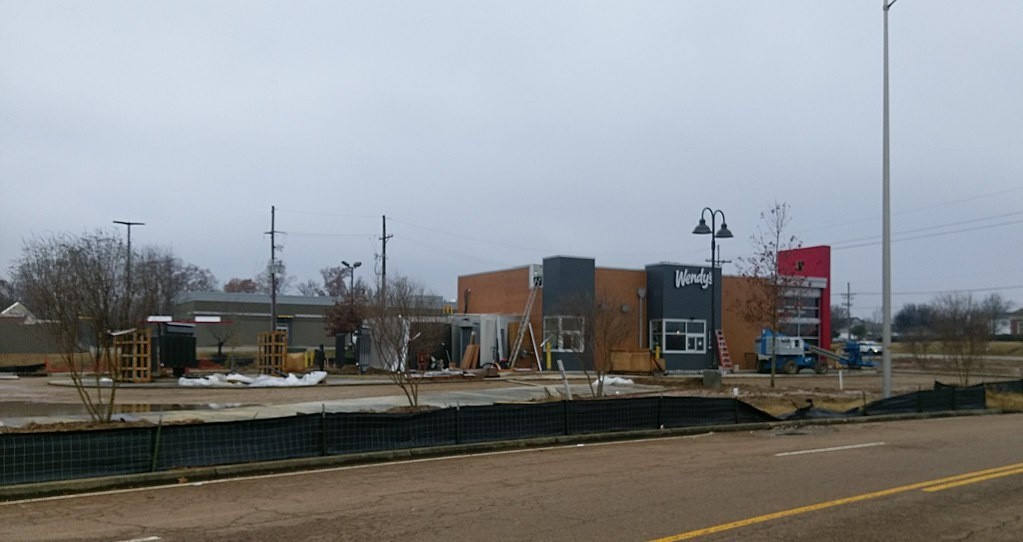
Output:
[857,340,883,357]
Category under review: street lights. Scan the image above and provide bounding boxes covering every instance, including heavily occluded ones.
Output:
[341,260,362,346]
[692,206,733,371]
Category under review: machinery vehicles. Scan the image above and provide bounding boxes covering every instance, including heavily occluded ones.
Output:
[753,328,881,376]
